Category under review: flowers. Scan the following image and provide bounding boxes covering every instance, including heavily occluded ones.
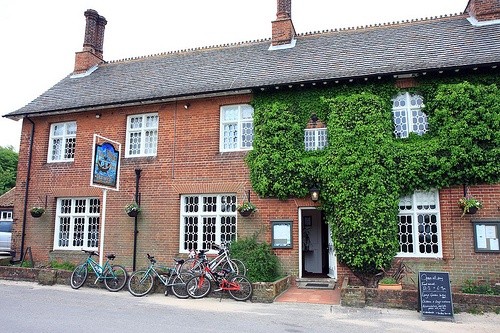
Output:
[237,200,256,214]
[124,203,142,215]
[28,206,45,214]
[456,196,483,218]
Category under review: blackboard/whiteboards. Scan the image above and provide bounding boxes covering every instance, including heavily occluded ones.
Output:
[418,271,453,316]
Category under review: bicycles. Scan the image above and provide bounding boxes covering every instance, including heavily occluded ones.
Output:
[70,250,128,292]
[128,253,198,299]
[176,239,254,301]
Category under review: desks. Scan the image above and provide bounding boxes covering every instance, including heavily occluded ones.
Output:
[302,250,313,276]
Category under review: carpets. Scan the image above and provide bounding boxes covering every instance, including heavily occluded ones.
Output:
[306,283,329,287]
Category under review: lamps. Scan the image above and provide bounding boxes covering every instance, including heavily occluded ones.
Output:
[310,185,320,204]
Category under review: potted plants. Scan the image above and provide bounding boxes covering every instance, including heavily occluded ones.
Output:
[378,276,403,290]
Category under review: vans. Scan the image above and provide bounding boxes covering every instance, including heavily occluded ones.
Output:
[0,219,13,255]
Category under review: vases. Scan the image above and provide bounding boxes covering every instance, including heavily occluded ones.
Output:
[127,210,138,217]
[239,210,253,217]
[31,212,43,217]
[462,206,477,214]
